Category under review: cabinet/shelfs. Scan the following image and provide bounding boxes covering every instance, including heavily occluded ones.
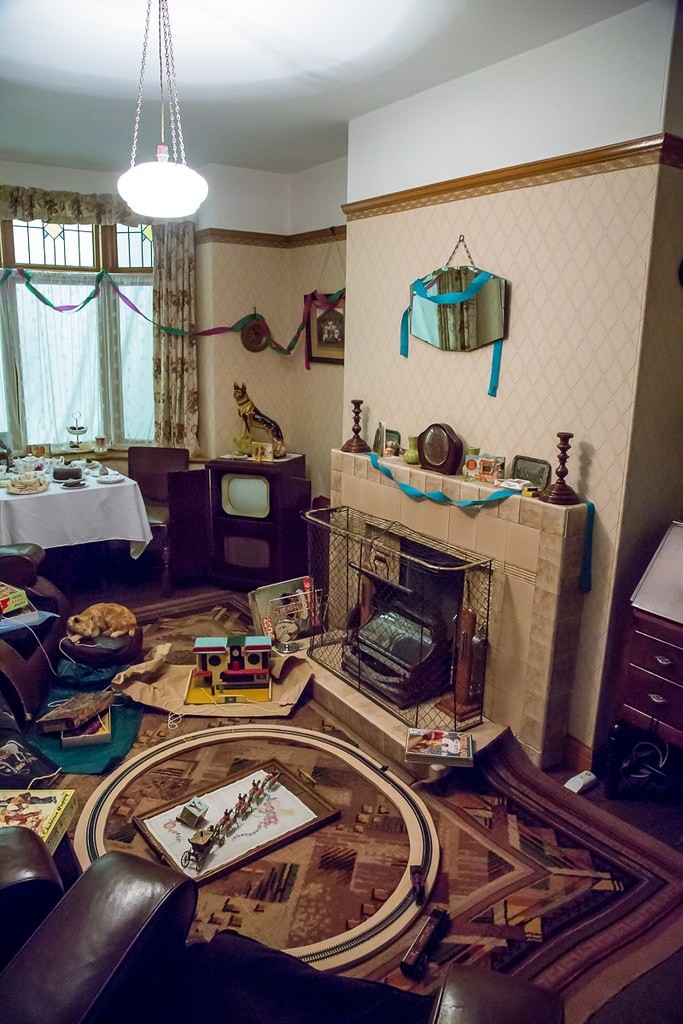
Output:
[601,601,682,807]
[164,452,314,593]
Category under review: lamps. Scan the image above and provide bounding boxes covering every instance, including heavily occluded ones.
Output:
[117,0,209,219]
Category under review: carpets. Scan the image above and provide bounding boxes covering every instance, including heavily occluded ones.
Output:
[0,591,683,1023]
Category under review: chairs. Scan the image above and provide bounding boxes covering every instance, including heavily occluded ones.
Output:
[92,446,196,597]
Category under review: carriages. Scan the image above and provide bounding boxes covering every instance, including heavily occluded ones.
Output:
[180,767,283,872]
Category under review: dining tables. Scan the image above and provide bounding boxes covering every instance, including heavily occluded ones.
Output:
[0,458,153,594]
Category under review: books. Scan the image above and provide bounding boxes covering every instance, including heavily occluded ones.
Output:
[404,726,474,768]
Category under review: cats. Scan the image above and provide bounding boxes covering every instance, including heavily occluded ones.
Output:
[66,602,137,639]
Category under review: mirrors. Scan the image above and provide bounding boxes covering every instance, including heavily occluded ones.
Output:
[409,264,505,353]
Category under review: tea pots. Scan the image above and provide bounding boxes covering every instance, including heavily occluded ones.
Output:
[18,453,46,473]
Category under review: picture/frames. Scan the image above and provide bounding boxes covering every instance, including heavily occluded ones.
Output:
[303,293,344,366]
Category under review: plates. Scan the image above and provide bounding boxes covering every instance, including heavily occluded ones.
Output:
[61,483,90,489]
[82,461,103,469]
[96,476,125,484]
[6,484,48,496]
[93,471,120,478]
[51,476,85,483]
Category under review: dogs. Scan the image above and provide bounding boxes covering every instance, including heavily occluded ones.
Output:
[233,382,287,458]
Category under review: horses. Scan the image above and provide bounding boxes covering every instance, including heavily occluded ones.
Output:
[215,769,282,836]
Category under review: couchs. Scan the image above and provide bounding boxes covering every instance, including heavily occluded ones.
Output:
[0,817,84,974]
[0,542,72,733]
[0,850,569,1024]
